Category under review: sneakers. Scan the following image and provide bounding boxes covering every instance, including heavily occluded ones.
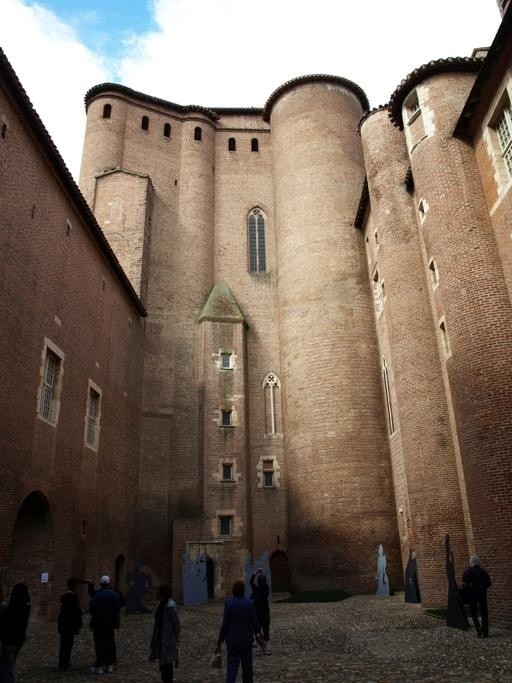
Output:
[91,667,104,674]
[108,665,112,673]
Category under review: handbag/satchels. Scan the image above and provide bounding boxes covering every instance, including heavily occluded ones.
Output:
[209,651,222,669]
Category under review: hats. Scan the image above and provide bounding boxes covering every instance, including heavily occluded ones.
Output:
[101,576,110,583]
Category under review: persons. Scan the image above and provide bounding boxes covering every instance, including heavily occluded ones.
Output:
[0,580,33,680]
[463,555,494,638]
[87,573,122,674]
[55,577,84,668]
[148,585,180,682]
[88,576,127,666]
[214,577,266,683]
[249,567,271,656]
